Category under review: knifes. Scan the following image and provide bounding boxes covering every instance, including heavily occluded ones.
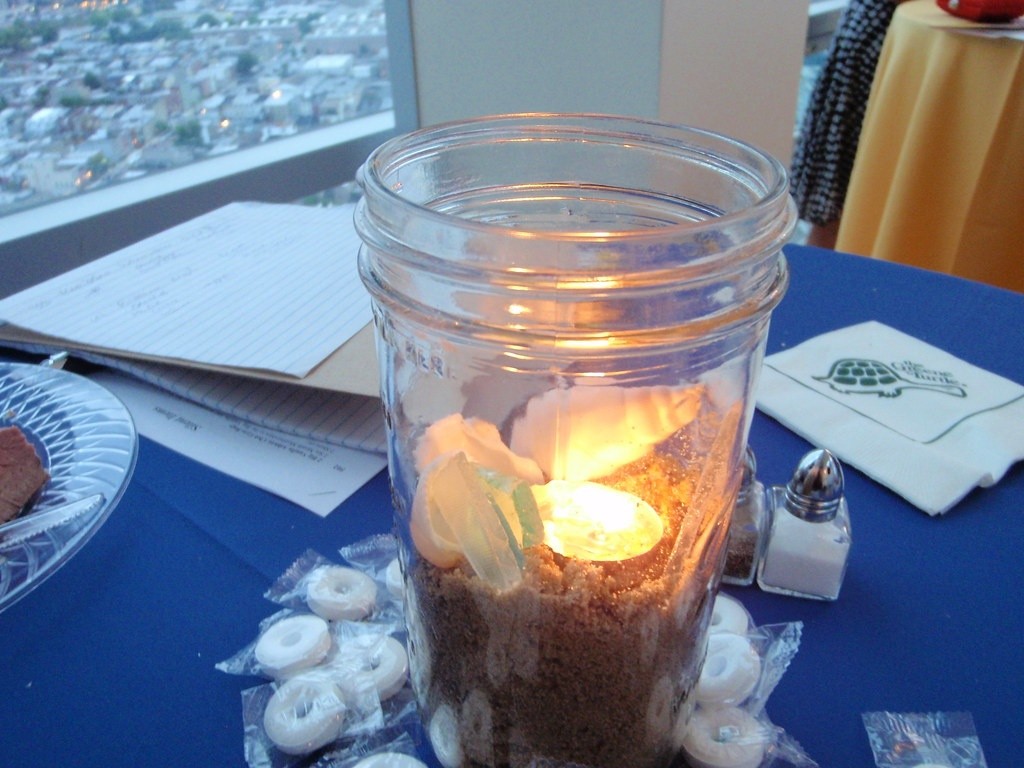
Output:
[1,492,103,553]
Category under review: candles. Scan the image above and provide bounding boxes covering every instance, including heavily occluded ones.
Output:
[355,112,798,768]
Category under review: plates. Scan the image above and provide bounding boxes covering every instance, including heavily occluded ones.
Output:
[0,356,144,620]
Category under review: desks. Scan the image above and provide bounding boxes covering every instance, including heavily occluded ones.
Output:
[0,180,1024,768]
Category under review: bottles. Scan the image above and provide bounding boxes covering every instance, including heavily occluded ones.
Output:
[754,449,853,599]
[718,440,765,587]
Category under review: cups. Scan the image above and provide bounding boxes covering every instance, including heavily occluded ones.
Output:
[358,116,794,768]
